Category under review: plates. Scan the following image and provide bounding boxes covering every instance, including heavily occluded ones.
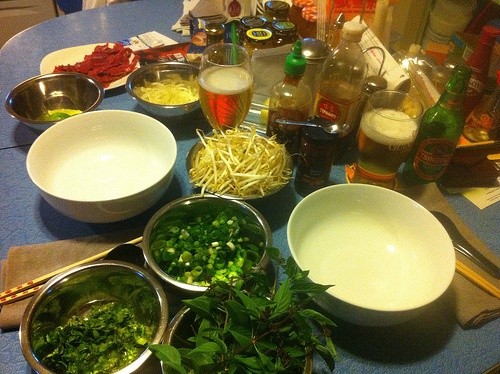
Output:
[40,43,141,90]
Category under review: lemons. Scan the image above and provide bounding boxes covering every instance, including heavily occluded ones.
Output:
[44,108,83,121]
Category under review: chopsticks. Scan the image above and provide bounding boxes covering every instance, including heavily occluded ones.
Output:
[0,236,143,305]
[455,259,500,298]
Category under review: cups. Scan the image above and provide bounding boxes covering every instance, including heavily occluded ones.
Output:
[198,43,253,130]
[346,89,424,191]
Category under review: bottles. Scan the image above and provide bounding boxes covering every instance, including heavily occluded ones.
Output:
[313,21,367,131]
[349,75,388,136]
[457,70,500,145]
[266,39,312,166]
[403,64,472,184]
[463,25,500,123]
[295,119,339,198]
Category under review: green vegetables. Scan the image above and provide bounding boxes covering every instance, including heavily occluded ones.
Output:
[32,301,152,374]
[147,247,336,374]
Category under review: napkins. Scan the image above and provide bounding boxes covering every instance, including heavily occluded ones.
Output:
[0,228,143,329]
[393,182,500,329]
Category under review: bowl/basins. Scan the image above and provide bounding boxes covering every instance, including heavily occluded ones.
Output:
[287,183,456,326]
[160,294,313,374]
[26,109,178,223]
[19,260,169,374]
[143,194,273,292]
[126,61,201,117]
[186,129,294,203]
[5,72,105,130]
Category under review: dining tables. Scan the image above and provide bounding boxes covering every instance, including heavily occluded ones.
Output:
[0,0,500,374]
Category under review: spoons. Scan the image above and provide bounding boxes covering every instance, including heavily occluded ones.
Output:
[430,212,500,279]
[276,118,352,134]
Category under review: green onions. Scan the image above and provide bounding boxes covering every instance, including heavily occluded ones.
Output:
[154,217,263,286]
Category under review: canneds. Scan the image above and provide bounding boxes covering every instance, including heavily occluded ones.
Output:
[295,118,336,198]
[235,0,297,60]
